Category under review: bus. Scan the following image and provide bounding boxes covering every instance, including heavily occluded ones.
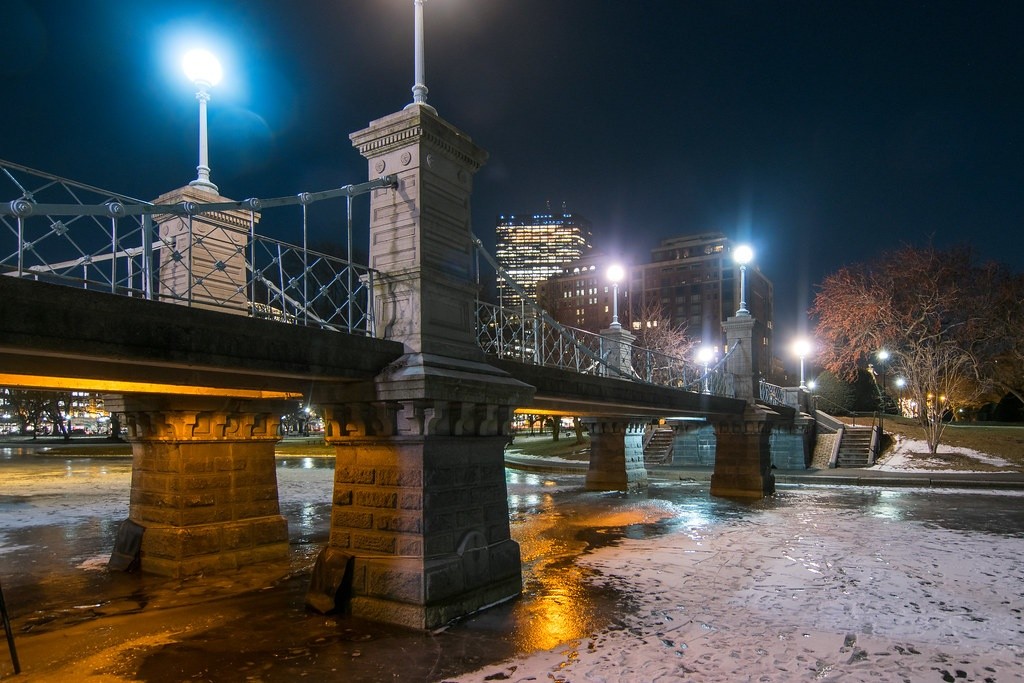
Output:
[510,413,545,429]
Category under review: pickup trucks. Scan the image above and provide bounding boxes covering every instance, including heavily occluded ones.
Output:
[71,429,86,434]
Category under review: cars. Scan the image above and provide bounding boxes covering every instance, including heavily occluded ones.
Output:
[313,426,320,431]
[1,430,10,435]
[120,429,128,434]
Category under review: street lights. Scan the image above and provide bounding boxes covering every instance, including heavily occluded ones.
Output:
[796,342,808,390]
[897,380,903,416]
[733,246,752,317]
[305,408,309,437]
[182,49,221,195]
[607,265,624,328]
[699,350,712,395]
[33,417,38,439]
[808,382,815,393]
[879,352,888,414]
